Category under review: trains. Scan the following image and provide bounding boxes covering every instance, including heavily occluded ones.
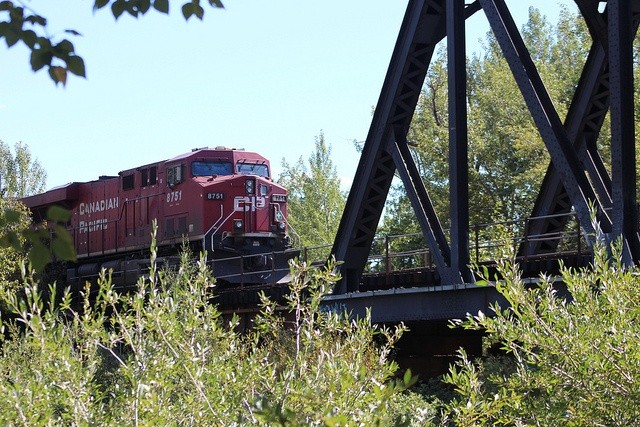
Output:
[0,146,301,307]
